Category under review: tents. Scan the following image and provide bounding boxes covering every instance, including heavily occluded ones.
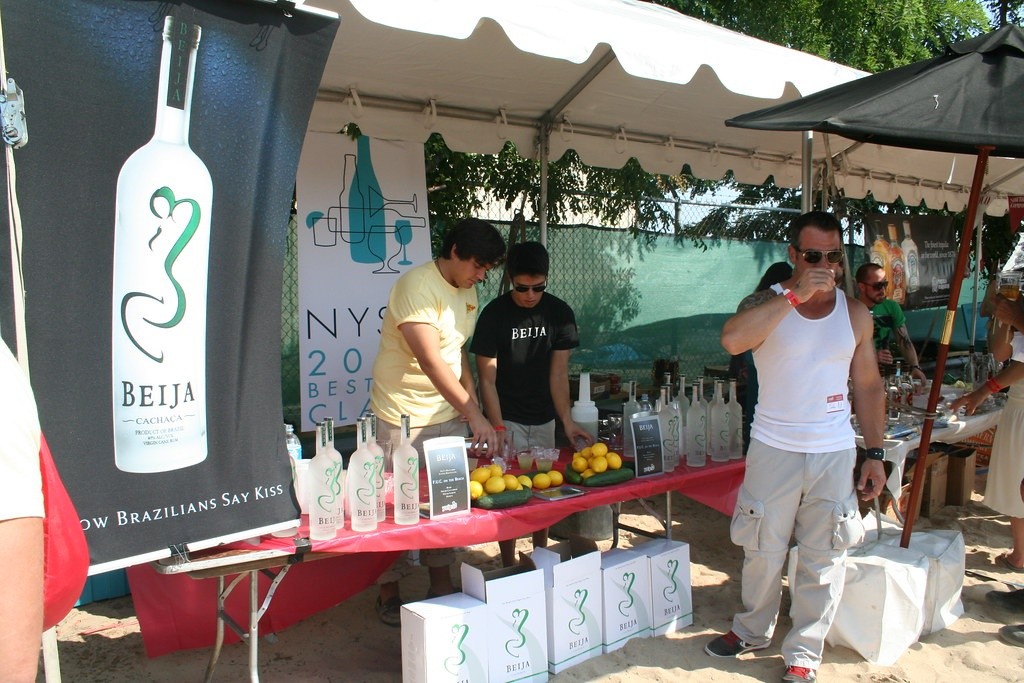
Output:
[293,0,1023,348]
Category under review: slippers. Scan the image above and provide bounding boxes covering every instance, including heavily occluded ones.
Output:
[997,553,1024,573]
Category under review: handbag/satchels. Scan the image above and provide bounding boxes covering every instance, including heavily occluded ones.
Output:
[40,434,90,633]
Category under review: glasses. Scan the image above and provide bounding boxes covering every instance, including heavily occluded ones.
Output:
[797,246,844,264]
[865,280,888,291]
[512,280,547,293]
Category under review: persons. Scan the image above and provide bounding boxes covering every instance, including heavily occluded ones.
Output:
[468,240,596,570]
[0,331,45,680]
[704,212,888,683]
[985,477,1024,648]
[949,250,1024,573]
[727,262,794,456]
[855,263,927,385]
[371,217,506,624]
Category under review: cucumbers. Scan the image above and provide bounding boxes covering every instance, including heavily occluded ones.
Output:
[474,471,548,509]
[564,460,634,487]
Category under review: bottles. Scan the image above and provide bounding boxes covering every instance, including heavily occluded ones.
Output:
[308,422,337,540]
[392,413,419,525]
[870,220,891,300]
[323,416,344,530]
[639,393,652,411]
[962,346,997,410]
[112,14,214,475]
[655,372,708,473]
[284,424,303,461]
[349,416,378,532]
[571,371,598,443]
[726,379,743,459]
[271,454,298,538]
[711,380,730,462]
[881,360,913,423]
[708,377,720,456]
[623,381,641,457]
[901,220,921,293]
[886,224,906,305]
[365,413,386,523]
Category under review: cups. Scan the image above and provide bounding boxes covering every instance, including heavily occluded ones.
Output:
[608,413,624,451]
[492,429,562,473]
[997,270,1022,301]
[913,379,933,396]
[574,436,595,452]
[598,420,608,441]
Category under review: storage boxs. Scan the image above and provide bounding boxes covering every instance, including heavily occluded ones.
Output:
[873,443,977,526]
[400,538,693,683]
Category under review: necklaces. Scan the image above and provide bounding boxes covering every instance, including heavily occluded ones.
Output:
[871,303,874,307]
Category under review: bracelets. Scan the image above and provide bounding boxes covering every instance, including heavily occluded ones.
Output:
[783,288,800,307]
[495,425,507,432]
[985,378,1003,393]
[910,365,922,373]
[866,447,887,462]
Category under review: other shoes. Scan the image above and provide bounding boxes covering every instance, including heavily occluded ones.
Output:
[425,589,460,599]
[376,596,404,624]
[999,625,1024,648]
[987,590,1024,614]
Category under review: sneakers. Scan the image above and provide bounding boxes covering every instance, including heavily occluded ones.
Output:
[705,630,770,658]
[781,665,817,683]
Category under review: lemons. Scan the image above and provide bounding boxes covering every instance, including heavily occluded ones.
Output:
[572,443,623,478]
[468,463,564,501]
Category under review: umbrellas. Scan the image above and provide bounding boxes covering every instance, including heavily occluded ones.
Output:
[725,26,1024,548]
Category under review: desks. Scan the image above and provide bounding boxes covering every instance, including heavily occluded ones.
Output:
[153,372,1012,683]
